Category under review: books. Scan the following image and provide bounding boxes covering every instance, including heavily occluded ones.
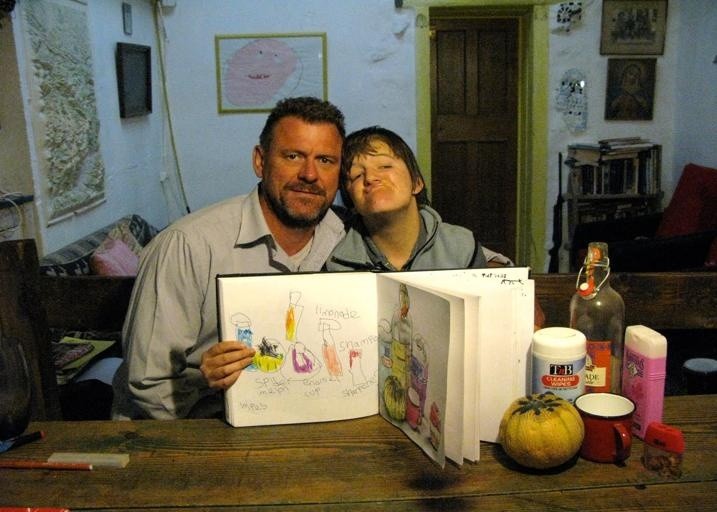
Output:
[564,135,662,195]
[212,262,536,469]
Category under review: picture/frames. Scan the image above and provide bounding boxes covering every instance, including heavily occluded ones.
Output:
[599,0,669,119]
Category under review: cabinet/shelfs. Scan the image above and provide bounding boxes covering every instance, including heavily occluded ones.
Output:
[549,154,665,272]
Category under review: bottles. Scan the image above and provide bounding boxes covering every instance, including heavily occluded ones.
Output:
[568,242,625,396]
[532,326,587,404]
[619,326,669,437]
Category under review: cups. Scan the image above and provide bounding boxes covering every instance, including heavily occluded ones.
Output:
[572,393,636,463]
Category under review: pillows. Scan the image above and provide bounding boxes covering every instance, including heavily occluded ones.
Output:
[90,214,143,278]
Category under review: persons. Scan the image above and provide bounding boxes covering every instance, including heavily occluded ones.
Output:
[320,123,512,274]
[108,97,349,420]
[609,60,648,111]
[613,7,654,40]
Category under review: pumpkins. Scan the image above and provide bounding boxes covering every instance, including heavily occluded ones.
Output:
[497,394,587,472]
[383,376,407,423]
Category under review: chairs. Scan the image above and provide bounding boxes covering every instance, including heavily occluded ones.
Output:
[574,163,716,270]
[38,214,159,278]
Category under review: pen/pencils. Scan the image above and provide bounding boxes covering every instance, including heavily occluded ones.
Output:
[0,430,47,453]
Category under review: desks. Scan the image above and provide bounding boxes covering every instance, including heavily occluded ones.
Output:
[0,393,714,512]
